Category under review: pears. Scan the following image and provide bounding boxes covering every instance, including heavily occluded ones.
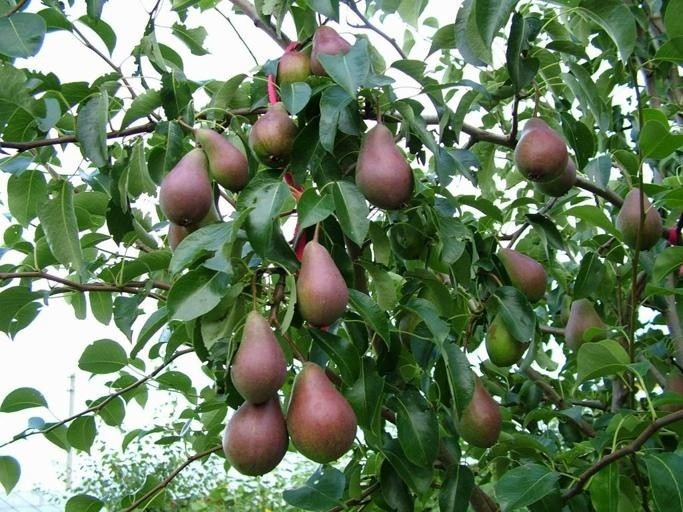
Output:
[159,25,683,476]
